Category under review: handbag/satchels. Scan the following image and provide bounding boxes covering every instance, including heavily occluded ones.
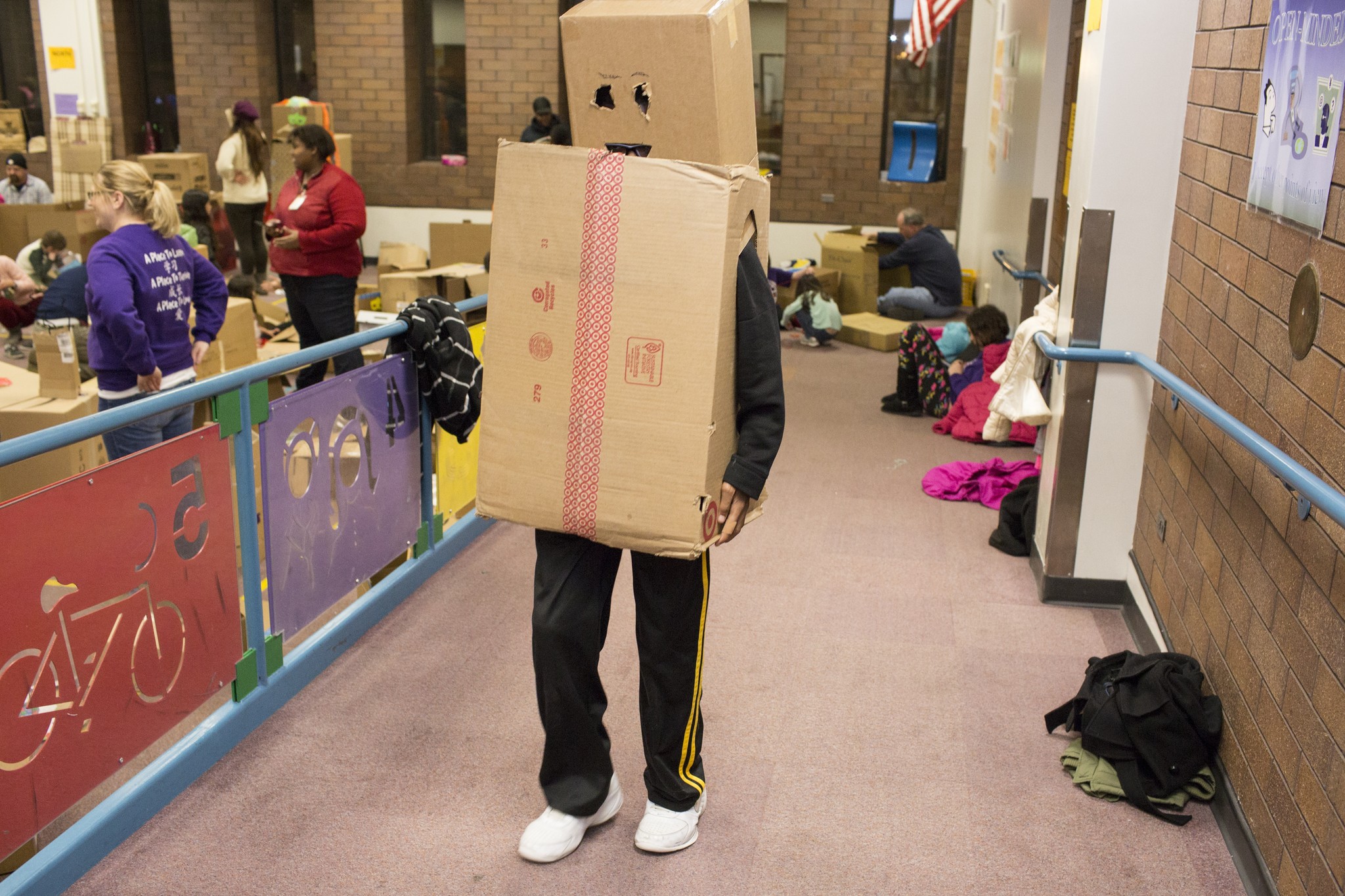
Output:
[32,311,81,400]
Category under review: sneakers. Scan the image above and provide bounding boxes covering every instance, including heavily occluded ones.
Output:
[634,788,706,853]
[518,773,625,863]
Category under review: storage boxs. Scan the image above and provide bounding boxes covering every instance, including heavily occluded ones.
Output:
[834,311,911,353]
[772,268,843,323]
[1,99,490,504]
[813,226,912,316]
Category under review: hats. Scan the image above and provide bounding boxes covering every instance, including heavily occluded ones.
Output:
[532,96,551,116]
[233,102,259,122]
[5,154,26,169]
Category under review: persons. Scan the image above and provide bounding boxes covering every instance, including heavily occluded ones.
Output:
[867,207,962,321]
[0,230,95,383]
[180,175,236,272]
[475,0,785,863]
[215,101,269,295]
[84,159,228,461]
[780,273,843,347]
[520,97,573,148]
[880,304,1012,417]
[0,152,55,204]
[263,124,366,391]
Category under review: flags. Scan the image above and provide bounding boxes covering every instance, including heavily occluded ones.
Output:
[905,0,968,70]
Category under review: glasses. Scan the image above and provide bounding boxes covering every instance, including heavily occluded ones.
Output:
[87,191,134,210]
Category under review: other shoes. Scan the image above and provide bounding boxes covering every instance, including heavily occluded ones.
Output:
[880,393,922,417]
[4,343,24,359]
[233,272,258,285]
[801,337,818,347]
